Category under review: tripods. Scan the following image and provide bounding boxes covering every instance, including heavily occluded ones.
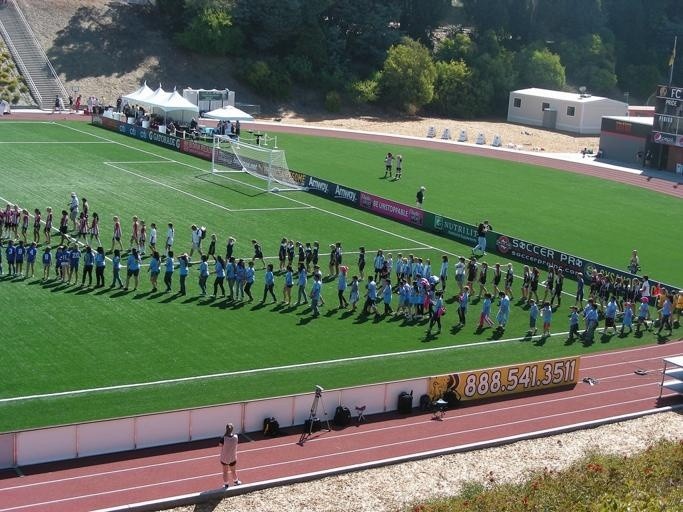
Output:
[298,393,330,444]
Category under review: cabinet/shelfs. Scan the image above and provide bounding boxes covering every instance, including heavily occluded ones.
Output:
[655,355,683,407]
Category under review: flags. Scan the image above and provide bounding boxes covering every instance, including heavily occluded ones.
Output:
[667,38,677,66]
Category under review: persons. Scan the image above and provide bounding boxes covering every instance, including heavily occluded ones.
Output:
[568,269,683,344]
[394,155,402,179]
[188,224,266,300]
[260,238,365,316]
[0,204,53,280]
[629,250,639,274]
[634,149,656,169]
[384,152,395,177]
[522,266,564,337]
[117,96,122,113]
[217,120,240,137]
[455,258,514,332]
[580,145,608,161]
[361,249,448,333]
[472,220,489,256]
[55,192,124,287]
[124,103,176,133]
[125,216,189,296]
[52,95,104,114]
[416,186,425,207]
[218,423,243,489]
[190,118,198,132]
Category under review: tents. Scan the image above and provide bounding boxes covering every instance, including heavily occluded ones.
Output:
[123,81,200,127]
